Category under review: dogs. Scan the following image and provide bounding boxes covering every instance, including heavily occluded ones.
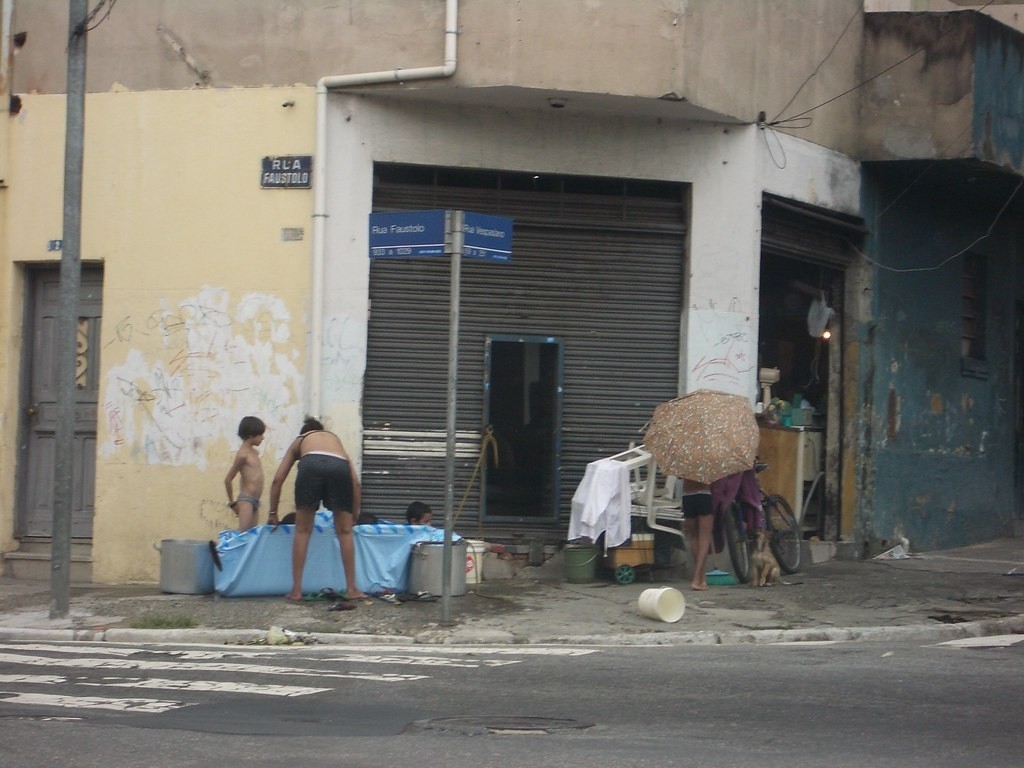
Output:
[749,529,804,587]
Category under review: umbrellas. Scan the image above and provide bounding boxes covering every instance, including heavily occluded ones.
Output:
[641,389,762,485]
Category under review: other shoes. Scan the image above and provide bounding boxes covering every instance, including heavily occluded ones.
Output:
[408,592,438,601]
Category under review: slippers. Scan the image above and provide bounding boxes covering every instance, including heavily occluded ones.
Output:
[319,587,342,599]
[379,593,397,602]
[303,592,329,601]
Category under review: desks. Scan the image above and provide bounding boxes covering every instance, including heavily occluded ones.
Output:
[756,422,826,528]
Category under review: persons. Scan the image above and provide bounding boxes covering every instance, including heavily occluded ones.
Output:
[677,477,715,590]
[225,416,265,534]
[406,502,432,525]
[356,513,377,525]
[267,420,366,600]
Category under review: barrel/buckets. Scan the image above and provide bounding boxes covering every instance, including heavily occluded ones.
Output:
[564,545,602,584]
[151,539,214,595]
[637,587,687,624]
[410,539,469,598]
[457,539,487,583]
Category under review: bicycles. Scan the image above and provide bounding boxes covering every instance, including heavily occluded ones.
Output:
[721,458,801,583]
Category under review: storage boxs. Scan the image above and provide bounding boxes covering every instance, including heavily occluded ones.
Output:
[604,548,655,569]
[608,532,655,549]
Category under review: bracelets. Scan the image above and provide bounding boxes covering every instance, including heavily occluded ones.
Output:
[268,511,277,514]
